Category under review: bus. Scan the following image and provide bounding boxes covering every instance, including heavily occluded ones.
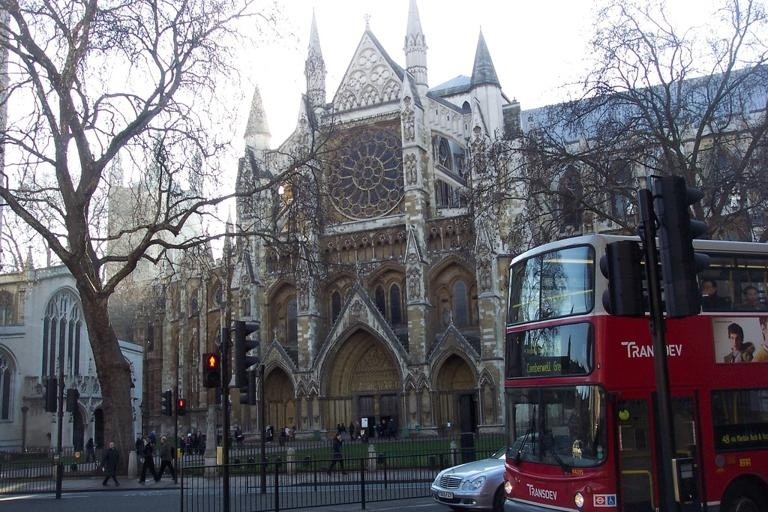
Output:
[502,234,767,511]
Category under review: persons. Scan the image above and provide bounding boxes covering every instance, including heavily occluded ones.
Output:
[285,426,296,440]
[102,442,120,484]
[180,433,206,456]
[752,316,768,363]
[337,418,398,442]
[135,430,175,484]
[87,438,97,462]
[327,433,348,476]
[738,286,768,311]
[701,278,731,311]
[235,427,244,449]
[723,322,755,362]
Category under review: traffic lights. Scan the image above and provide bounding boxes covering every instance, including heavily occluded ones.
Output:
[598,241,643,317]
[159,390,172,417]
[235,320,259,388]
[66,388,79,412]
[203,353,219,388]
[656,174,710,320]
[177,399,186,415]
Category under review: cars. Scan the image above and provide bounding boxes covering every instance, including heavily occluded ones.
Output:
[430,445,506,511]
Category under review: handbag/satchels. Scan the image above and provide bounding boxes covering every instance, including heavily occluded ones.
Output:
[171,446,175,458]
